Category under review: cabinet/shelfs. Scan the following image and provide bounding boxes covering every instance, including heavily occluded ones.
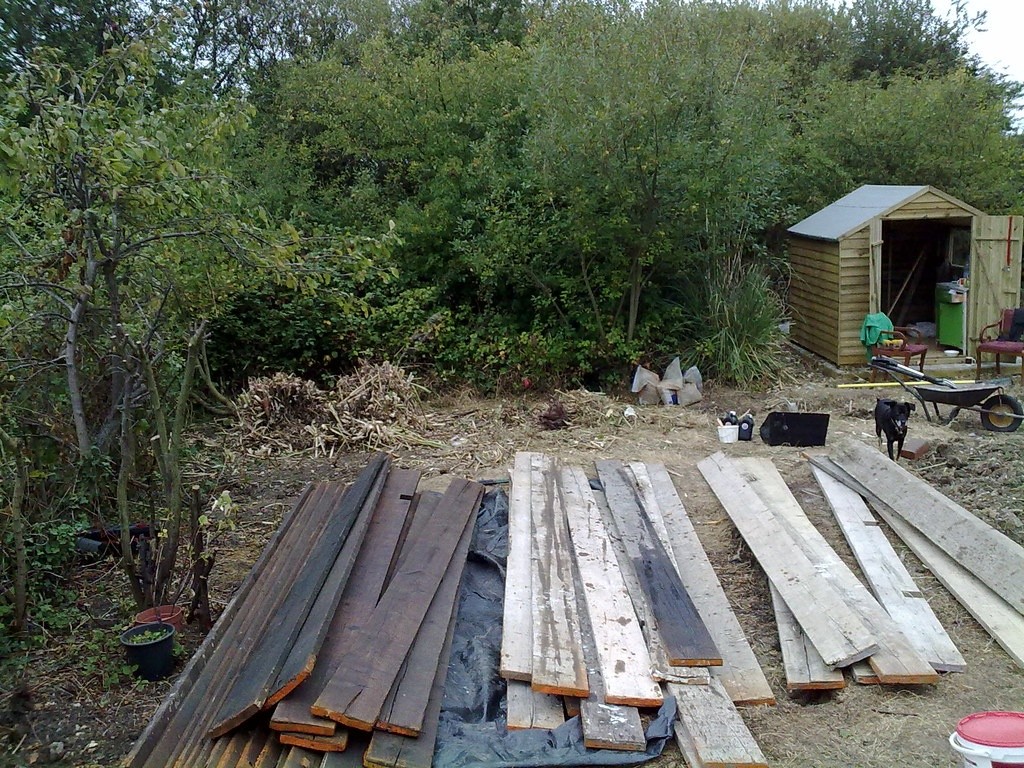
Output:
[934,286,970,355]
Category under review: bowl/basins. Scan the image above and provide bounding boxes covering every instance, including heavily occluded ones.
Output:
[944,350,959,357]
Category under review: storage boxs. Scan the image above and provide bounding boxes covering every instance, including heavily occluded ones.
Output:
[718,423,754,443]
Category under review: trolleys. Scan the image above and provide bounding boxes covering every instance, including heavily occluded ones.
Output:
[867,351,1024,433]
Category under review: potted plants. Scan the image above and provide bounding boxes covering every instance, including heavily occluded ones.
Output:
[133,450,240,636]
[119,618,176,681]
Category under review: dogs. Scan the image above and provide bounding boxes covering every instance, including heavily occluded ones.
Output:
[875,397,915,461]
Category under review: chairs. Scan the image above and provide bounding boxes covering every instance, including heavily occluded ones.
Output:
[976,309,1024,386]
[866,312,929,383]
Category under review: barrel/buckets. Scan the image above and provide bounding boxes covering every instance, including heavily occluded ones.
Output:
[949,710,1024,768]
[717,425,738,444]
[721,411,738,425]
[738,414,754,441]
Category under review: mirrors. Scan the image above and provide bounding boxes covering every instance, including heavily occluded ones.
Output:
[949,230,970,268]
